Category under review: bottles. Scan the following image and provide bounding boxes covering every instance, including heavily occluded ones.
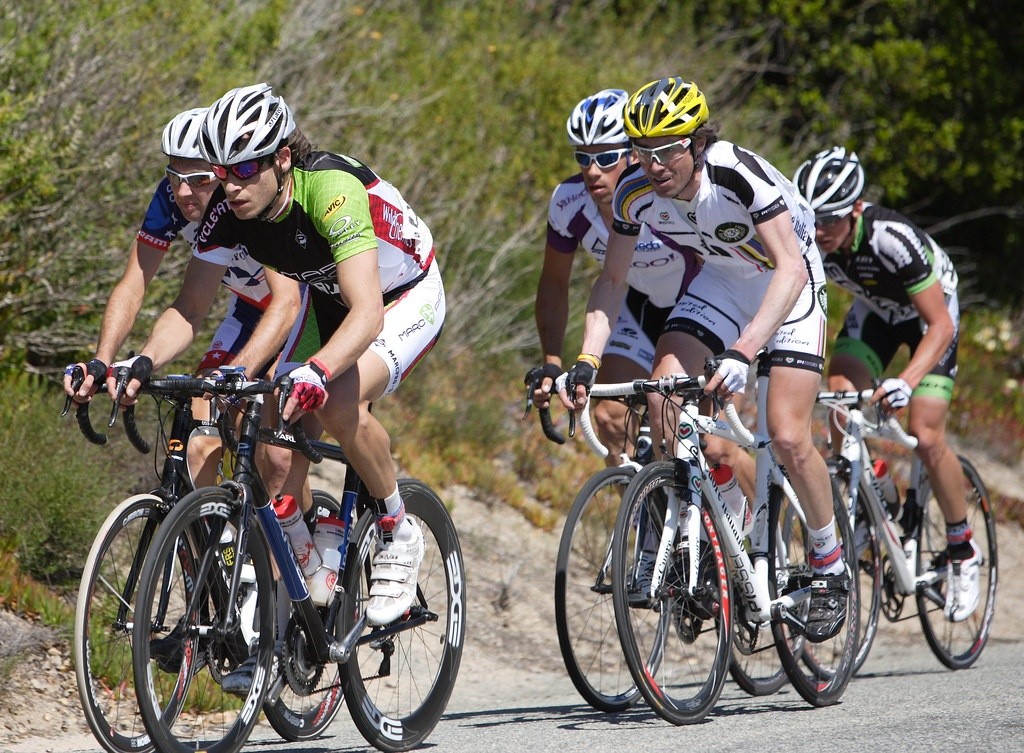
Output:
[272,493,322,581]
[205,516,257,585]
[869,458,899,505]
[305,511,346,608]
[710,457,756,536]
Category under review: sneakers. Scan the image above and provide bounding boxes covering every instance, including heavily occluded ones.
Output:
[630,549,658,599]
[365,511,426,626]
[667,542,713,589]
[851,508,904,561]
[222,640,284,693]
[148,615,206,664]
[943,539,987,622]
[804,561,854,643]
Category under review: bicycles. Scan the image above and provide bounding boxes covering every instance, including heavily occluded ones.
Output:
[107,365,468,753]
[520,356,1002,727]
[60,361,359,753]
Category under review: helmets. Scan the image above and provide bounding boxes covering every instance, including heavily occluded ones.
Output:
[197,83,296,166]
[792,145,865,212]
[161,108,209,159]
[565,88,632,147]
[621,76,710,138]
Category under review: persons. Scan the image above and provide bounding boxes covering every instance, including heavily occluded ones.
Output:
[64,107,316,672]
[108,81,448,694]
[525,88,759,602]
[555,76,853,642]
[790,144,981,621]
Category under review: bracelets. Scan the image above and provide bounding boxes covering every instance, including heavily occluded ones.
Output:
[307,357,330,382]
[577,353,602,369]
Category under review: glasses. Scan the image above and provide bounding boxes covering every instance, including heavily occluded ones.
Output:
[165,165,217,188]
[630,138,691,166]
[815,203,856,229]
[209,149,279,181]
[574,148,635,169]
